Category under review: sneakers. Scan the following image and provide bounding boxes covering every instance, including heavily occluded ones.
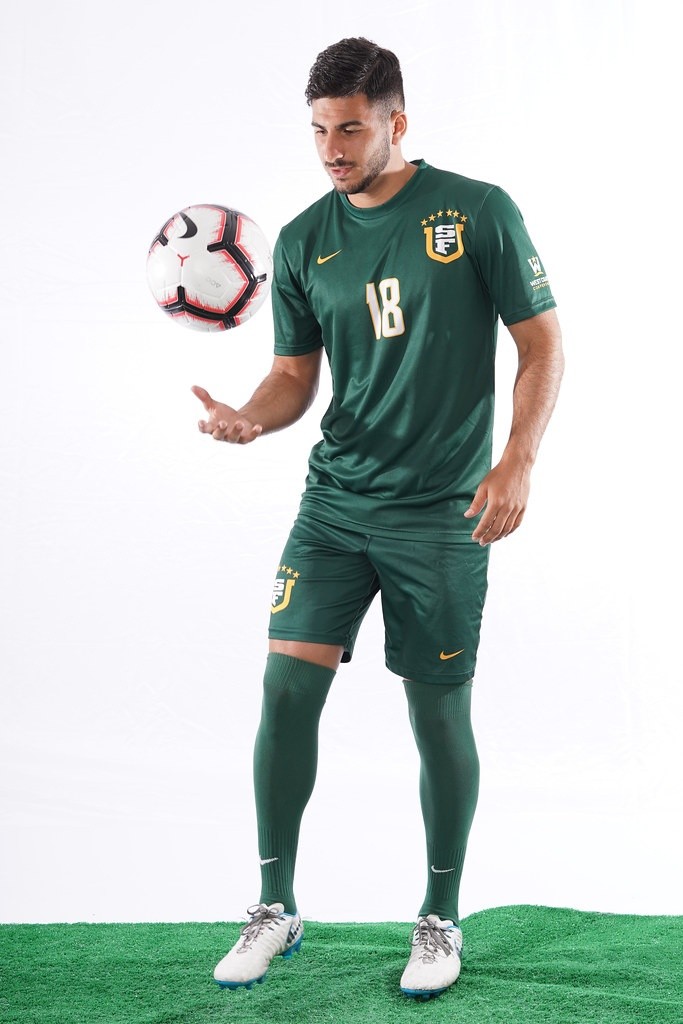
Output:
[399,914,462,1001]
[213,902,304,990]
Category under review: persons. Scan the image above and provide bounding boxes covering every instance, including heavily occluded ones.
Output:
[192,37,564,995]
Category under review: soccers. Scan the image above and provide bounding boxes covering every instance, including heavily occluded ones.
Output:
[151,204,274,332]
